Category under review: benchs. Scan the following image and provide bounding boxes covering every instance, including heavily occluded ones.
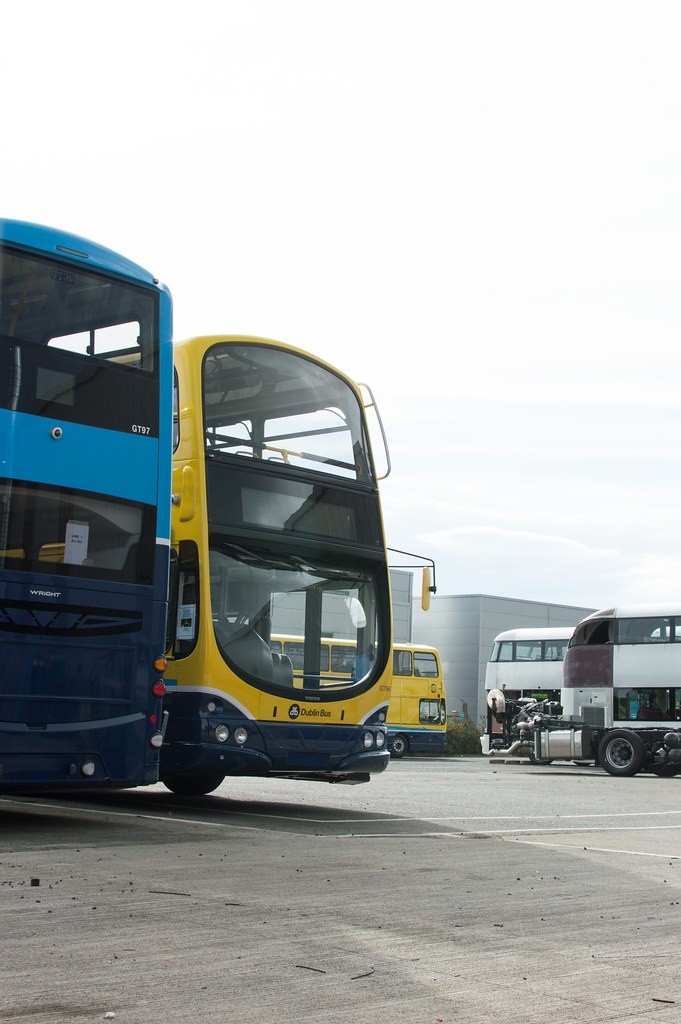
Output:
[619,635,666,642]
[636,704,664,720]
[401,667,420,677]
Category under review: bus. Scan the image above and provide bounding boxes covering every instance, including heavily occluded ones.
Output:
[479,606,681,774]
[269,633,447,758]
[0,333,437,795]
[1,218,173,787]
